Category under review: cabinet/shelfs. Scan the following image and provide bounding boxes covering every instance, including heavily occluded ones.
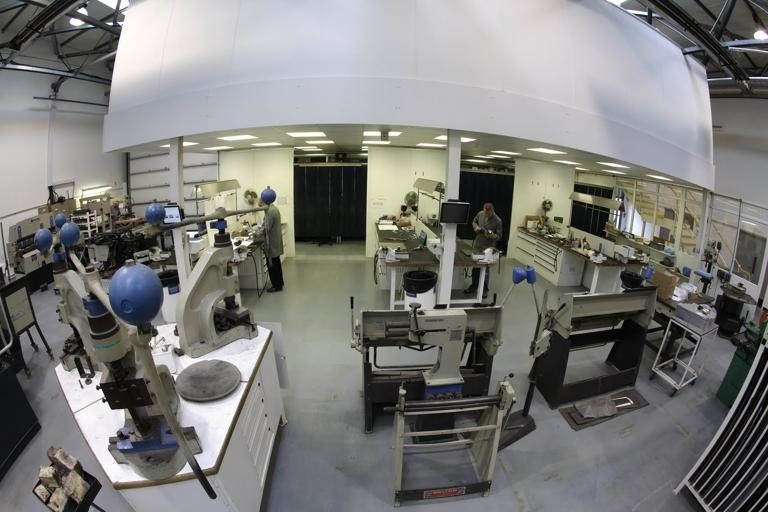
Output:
[71,210,99,240]
[54,321,291,512]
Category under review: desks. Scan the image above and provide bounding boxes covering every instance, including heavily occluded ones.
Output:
[0,273,54,376]
[422,219,495,304]
[231,224,288,289]
[375,223,440,310]
[517,227,622,293]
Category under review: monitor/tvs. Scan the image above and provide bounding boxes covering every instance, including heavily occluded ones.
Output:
[440,201,469,225]
[162,205,183,225]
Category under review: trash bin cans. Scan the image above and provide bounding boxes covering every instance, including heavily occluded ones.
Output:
[618,269,644,292]
[404,270,437,312]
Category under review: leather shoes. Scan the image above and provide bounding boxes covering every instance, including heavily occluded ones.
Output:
[464,284,478,293]
[482,288,489,298]
[266,287,282,292]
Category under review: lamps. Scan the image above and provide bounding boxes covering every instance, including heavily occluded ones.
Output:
[69,1,89,26]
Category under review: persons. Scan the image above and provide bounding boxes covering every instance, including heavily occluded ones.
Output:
[249,198,284,292]
[464,203,503,299]
[105,200,122,223]
